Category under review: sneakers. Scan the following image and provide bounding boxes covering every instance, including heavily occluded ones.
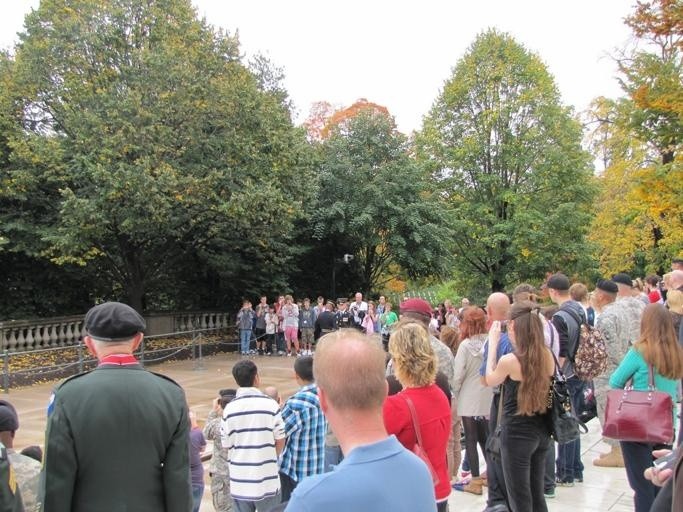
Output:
[573,476,583,482]
[541,485,556,497]
[454,470,472,484]
[552,472,574,487]
[240,349,313,357]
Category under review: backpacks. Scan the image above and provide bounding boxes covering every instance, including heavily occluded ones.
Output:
[572,319,608,382]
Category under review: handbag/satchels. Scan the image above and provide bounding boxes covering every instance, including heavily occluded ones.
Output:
[600,388,675,445]
[550,380,581,445]
[409,443,440,489]
[583,390,597,422]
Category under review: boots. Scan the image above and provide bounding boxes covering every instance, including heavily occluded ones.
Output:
[460,480,483,495]
[592,445,625,468]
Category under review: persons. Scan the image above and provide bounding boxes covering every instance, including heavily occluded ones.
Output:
[1,260,682,512]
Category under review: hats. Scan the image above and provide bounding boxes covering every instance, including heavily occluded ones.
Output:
[671,257,683,263]
[219,389,236,397]
[610,273,633,287]
[539,274,572,291]
[596,278,618,293]
[0,399,20,433]
[81,300,147,343]
[399,299,432,318]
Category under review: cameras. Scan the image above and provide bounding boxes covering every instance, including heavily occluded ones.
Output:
[497,322,510,333]
